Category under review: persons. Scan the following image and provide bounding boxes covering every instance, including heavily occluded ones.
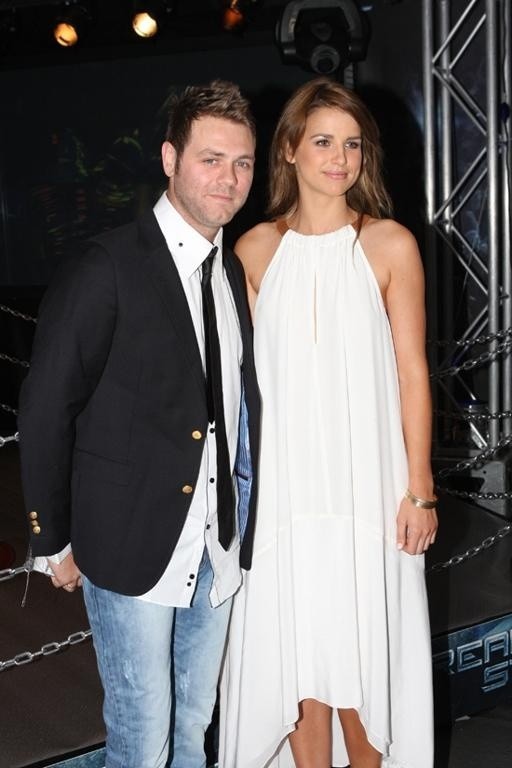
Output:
[16,71,261,767]
[232,76,443,767]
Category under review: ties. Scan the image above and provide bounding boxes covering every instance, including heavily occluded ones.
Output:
[200,246,237,552]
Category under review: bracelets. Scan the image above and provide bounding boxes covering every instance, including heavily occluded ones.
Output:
[402,489,440,513]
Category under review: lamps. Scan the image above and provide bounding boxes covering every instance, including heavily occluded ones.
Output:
[52,0,96,48]
[129,0,173,39]
